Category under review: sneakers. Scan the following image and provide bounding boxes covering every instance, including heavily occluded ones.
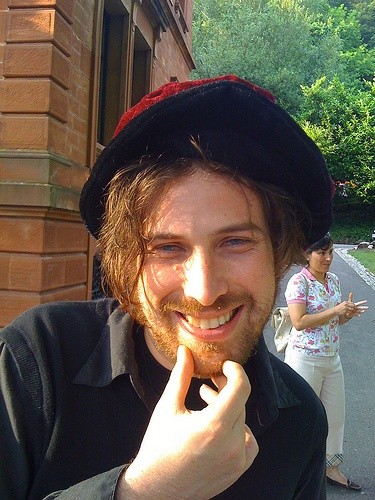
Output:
[327,474,361,490]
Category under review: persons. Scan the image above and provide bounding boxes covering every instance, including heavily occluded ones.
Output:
[0,76,335,500]
[284,231,367,492]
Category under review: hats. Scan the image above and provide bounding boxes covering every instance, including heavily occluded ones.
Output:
[79,75,335,253]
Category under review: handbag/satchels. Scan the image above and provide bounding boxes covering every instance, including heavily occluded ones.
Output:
[272,273,307,351]
